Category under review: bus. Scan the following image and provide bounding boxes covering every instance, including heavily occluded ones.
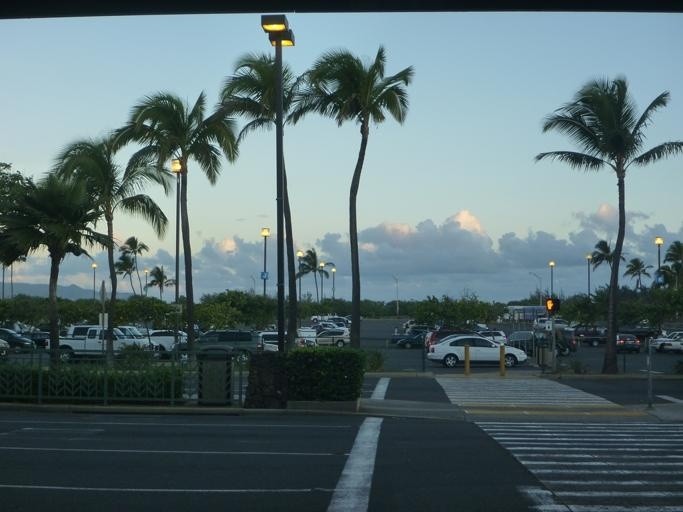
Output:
[497,305,548,325]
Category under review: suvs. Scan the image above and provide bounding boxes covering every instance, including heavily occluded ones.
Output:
[171,330,263,367]
[145,329,188,357]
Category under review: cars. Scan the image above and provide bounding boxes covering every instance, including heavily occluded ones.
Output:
[392,316,528,369]
[649,331,683,352]
[0,320,51,358]
[615,333,641,354]
[261,314,364,352]
[509,317,607,359]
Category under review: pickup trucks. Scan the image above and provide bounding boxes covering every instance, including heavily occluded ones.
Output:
[44,328,158,362]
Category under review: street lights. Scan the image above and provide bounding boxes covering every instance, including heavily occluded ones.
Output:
[319,261,324,302]
[296,250,303,326]
[330,268,335,311]
[585,254,592,296]
[91,263,96,300]
[549,261,554,297]
[258,227,270,297]
[144,269,148,297]
[654,236,663,277]
[258,13,296,360]
[170,159,183,347]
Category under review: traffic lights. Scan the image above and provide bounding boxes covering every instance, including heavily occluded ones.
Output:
[546,298,559,311]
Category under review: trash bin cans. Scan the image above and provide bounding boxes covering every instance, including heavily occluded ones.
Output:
[536,345,549,366]
[195,346,233,406]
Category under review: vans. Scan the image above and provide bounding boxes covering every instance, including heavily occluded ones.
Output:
[67,325,147,349]
